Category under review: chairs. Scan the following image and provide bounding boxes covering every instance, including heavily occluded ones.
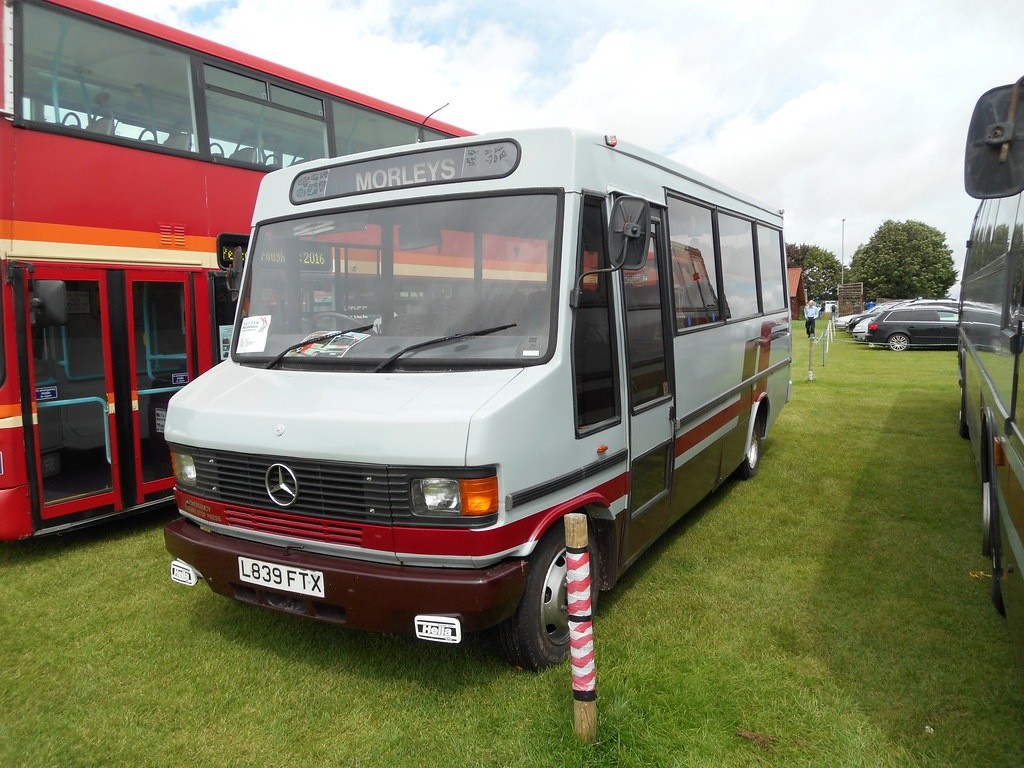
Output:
[61,105,310,168]
[384,291,536,337]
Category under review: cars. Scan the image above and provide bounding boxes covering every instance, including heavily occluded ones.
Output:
[835,296,958,352]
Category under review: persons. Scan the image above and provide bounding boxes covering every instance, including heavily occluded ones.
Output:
[817,301,837,320]
[804,299,819,338]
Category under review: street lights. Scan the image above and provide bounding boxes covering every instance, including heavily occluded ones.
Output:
[840,218,846,316]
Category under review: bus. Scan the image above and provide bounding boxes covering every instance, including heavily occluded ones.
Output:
[956,76,1024,695]
[166,127,794,676]
[1,1,718,543]
